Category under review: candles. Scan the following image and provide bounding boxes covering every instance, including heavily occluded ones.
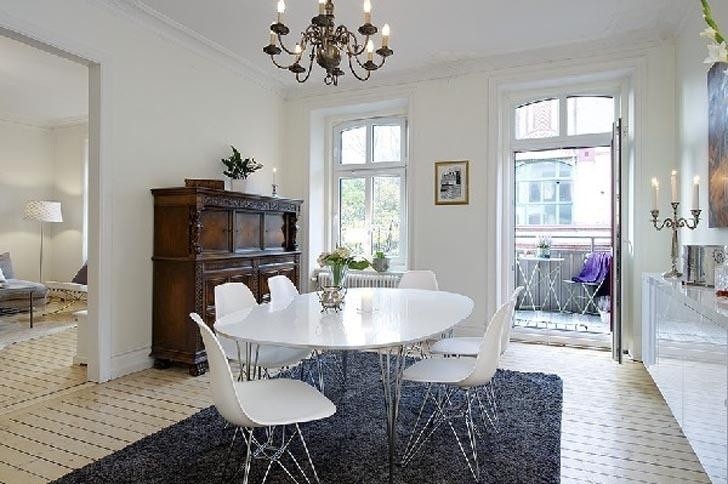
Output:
[273,168,276,183]
[650,171,699,209]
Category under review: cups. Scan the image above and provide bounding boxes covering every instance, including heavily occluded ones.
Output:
[715,266,728,298]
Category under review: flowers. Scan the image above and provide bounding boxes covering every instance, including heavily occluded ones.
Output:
[318,247,351,285]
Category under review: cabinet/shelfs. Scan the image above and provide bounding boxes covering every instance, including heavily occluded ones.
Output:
[148,187,305,376]
[641,274,728,484]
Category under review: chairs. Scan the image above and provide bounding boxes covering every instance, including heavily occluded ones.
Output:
[559,253,612,315]
[45,260,88,304]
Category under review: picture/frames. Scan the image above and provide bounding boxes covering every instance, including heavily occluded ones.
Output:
[435,160,470,205]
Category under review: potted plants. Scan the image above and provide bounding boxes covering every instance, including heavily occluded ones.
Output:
[221,144,264,192]
[372,251,391,271]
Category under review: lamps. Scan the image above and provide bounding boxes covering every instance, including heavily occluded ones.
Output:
[23,200,63,282]
[262,0,393,86]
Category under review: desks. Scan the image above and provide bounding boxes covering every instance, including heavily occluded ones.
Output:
[515,254,565,313]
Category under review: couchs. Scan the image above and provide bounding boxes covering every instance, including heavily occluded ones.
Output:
[0,252,47,312]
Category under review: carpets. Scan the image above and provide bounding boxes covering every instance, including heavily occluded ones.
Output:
[49,350,563,483]
